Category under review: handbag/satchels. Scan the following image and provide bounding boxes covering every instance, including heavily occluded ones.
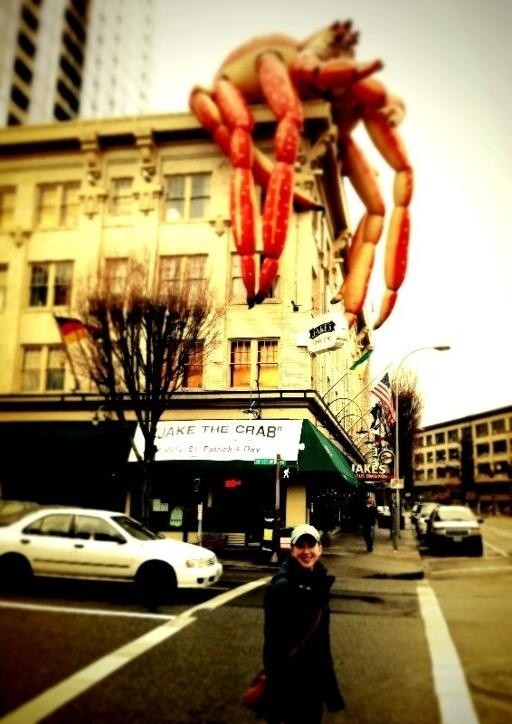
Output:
[241,668,266,707]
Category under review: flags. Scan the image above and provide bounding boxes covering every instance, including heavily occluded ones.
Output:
[372,373,394,417]
[54,317,98,345]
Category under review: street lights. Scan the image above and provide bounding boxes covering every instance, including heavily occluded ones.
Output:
[395,346,452,550]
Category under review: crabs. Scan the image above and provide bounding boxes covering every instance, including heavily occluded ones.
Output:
[188,19,415,331]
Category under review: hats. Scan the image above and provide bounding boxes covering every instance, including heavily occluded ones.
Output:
[290,524,321,545]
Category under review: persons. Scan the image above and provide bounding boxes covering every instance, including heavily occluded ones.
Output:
[262,525,347,724]
[360,496,381,552]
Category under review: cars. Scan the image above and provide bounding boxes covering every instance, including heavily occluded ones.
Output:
[410,502,484,555]
[0,506,223,600]
